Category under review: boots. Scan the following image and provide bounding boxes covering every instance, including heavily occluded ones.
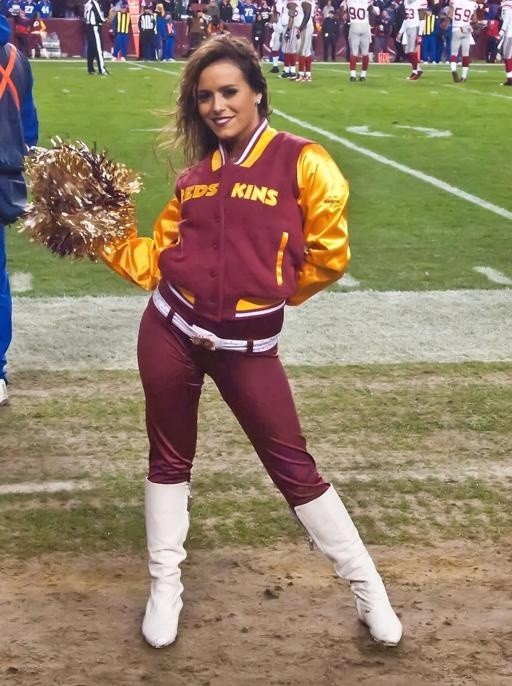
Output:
[294,482,403,647]
[143,477,192,650]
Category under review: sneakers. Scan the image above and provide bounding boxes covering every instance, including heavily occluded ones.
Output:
[501,81,510,85]
[351,76,366,80]
[267,66,312,82]
[453,69,466,82]
[408,68,423,80]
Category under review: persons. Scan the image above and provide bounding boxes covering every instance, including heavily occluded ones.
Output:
[0,1,512,85]
[96,32,403,651]
[0,15,40,406]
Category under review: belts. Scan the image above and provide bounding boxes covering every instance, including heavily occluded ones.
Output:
[153,287,279,353]
